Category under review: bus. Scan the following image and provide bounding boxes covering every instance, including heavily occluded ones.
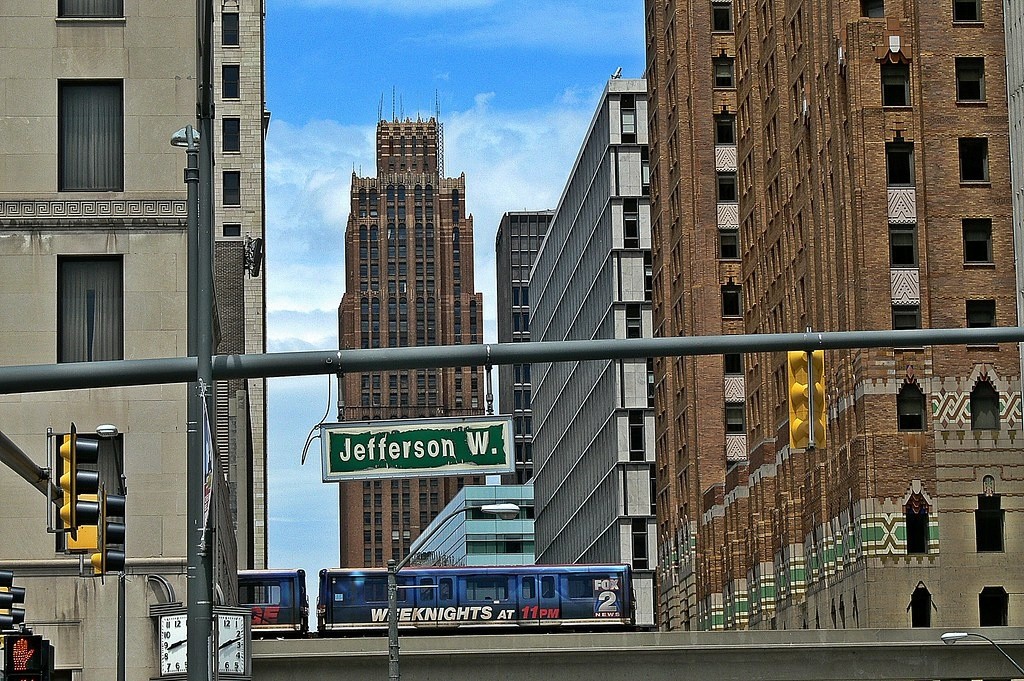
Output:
[316,565,637,638]
[238,569,308,640]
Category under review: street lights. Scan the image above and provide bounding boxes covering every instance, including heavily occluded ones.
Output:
[174,123,209,680]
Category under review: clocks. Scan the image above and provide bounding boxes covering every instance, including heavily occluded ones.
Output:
[211,611,248,678]
[158,612,188,678]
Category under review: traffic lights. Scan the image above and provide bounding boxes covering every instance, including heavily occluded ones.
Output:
[46,427,101,542]
[0,570,14,634]
[93,487,127,582]
[8,586,36,625]
